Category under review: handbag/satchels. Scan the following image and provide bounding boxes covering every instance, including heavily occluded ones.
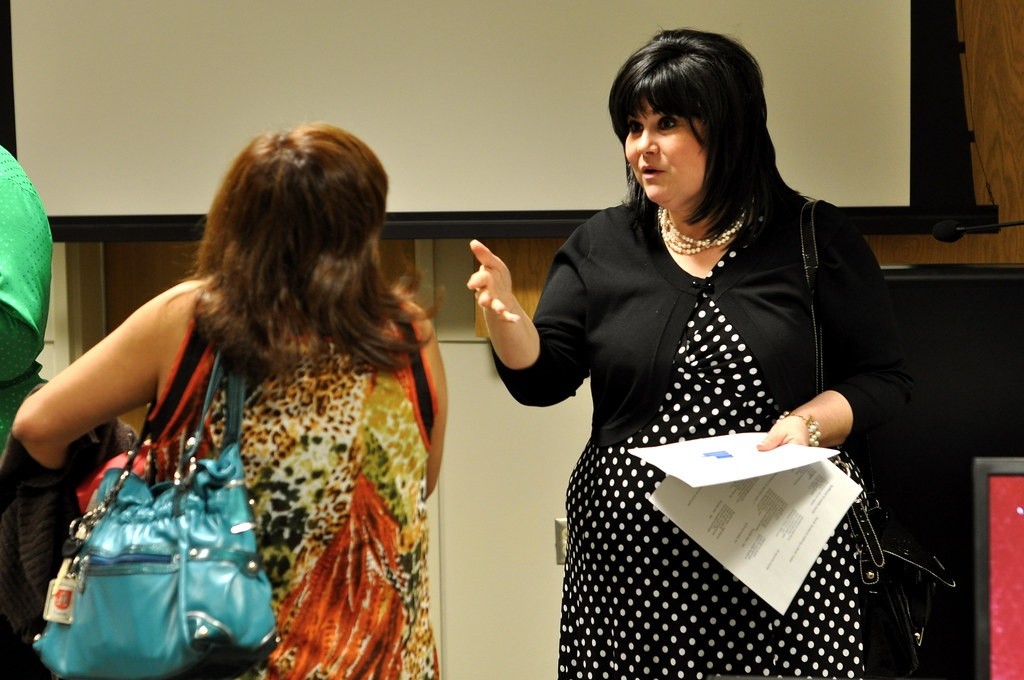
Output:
[855,517,956,680]
[30,342,280,680]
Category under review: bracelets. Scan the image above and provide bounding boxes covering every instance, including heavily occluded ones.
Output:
[777,410,821,447]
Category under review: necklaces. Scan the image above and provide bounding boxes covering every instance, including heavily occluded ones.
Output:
[654,204,747,257]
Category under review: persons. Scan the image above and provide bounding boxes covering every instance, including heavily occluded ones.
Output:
[466,29,905,680]
[11,124,447,680]
